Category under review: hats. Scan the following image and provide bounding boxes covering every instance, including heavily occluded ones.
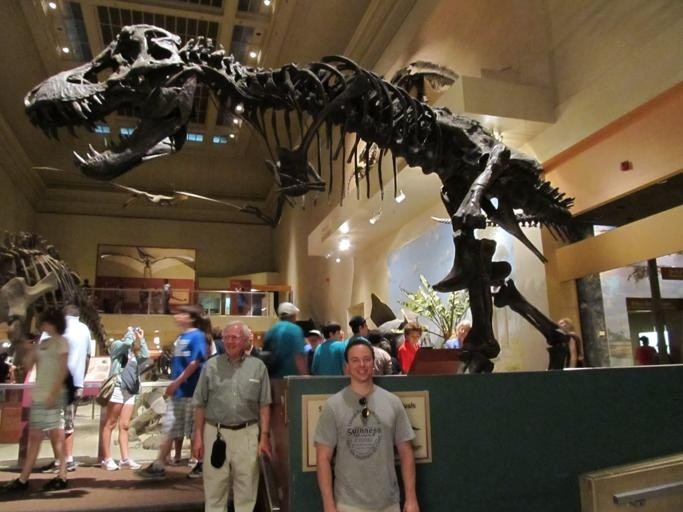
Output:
[305,330,321,338]
[349,316,368,327]
[279,302,300,317]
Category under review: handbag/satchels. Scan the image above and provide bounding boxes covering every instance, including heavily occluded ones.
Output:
[96,377,114,406]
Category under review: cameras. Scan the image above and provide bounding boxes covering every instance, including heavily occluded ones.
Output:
[133,328,140,332]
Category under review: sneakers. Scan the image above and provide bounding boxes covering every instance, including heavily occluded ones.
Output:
[1,478,28,493]
[142,463,167,476]
[41,461,77,473]
[120,459,141,471]
[101,459,119,471]
[174,457,204,478]
[43,477,68,490]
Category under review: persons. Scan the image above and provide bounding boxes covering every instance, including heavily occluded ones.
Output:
[444,320,470,374]
[80,279,91,306]
[0,304,91,497]
[245,301,422,379]
[234,287,245,314]
[313,339,420,512]
[159,279,172,316]
[99,326,151,471]
[110,286,124,313]
[191,320,273,512]
[133,303,224,482]
[634,336,657,365]
[558,318,583,369]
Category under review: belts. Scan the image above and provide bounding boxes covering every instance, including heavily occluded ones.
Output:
[216,421,256,429]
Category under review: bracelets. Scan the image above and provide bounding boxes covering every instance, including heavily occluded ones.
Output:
[260,431,271,438]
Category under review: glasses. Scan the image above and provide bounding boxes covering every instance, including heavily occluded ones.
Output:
[359,397,370,418]
